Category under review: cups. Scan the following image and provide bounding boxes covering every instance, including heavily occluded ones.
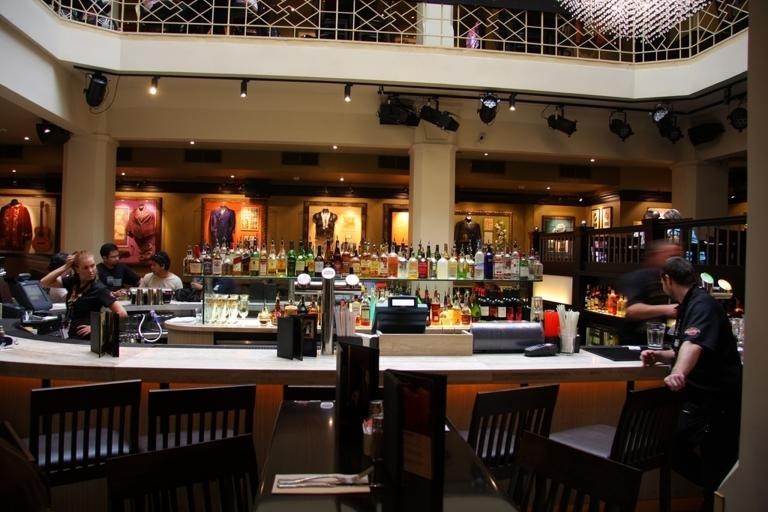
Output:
[647,321,666,349]
[560,333,576,356]
[120,292,128,300]
[257,313,270,325]
[729,317,744,341]
[135,287,164,305]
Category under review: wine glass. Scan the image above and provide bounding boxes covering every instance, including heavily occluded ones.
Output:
[532,296,542,313]
[204,293,249,326]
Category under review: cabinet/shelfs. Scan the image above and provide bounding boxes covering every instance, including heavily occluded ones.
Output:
[203,266,545,326]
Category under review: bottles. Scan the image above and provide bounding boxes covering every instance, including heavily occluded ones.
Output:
[271,293,321,326]
[0,325,6,339]
[118,334,137,344]
[183,236,544,279]
[340,286,531,326]
[151,312,175,330]
[587,285,627,317]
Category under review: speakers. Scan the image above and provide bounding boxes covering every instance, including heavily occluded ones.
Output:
[688,123,725,146]
[86,74,107,107]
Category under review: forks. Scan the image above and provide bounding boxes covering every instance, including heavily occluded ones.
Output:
[278,465,375,484]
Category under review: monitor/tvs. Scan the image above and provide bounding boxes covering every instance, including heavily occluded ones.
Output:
[16,280,54,311]
[371,303,427,332]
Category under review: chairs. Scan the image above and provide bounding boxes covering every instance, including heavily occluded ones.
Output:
[458,384,560,468]
[107,433,261,512]
[138,384,256,511]
[507,429,644,511]
[549,383,682,511]
[2,379,142,512]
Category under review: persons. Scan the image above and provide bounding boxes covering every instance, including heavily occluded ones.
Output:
[625,237,685,349]
[639,256,742,445]
[40,249,128,340]
[48,251,73,302]
[138,250,184,289]
[465,20,482,49]
[97,243,143,299]
[188,276,238,293]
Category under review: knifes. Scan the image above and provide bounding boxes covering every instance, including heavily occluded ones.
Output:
[276,483,383,488]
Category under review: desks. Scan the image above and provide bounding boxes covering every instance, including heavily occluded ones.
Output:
[252,384,521,511]
